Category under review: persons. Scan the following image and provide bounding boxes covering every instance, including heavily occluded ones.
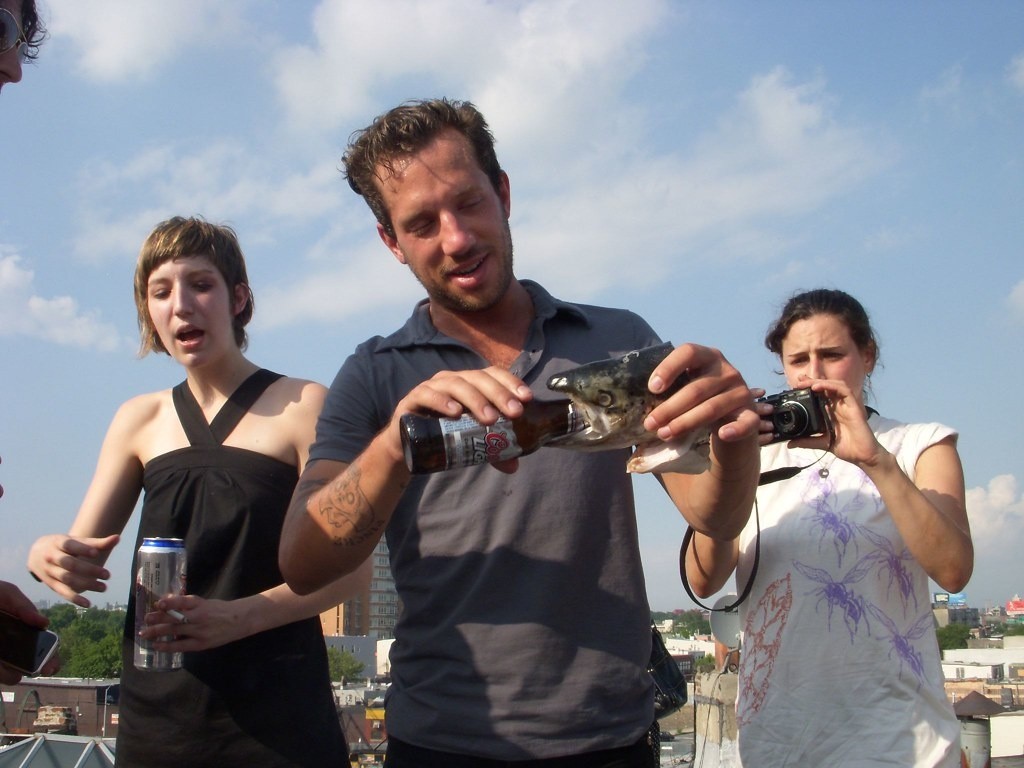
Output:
[0,0,58,687]
[679,289,977,768]
[276,95,767,768]
[26,215,377,768]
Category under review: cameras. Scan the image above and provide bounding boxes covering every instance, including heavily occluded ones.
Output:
[758,388,826,445]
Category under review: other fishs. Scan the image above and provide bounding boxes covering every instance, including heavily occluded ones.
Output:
[543,341,713,474]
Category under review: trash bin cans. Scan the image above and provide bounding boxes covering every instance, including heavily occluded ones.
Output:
[952,690,1007,768]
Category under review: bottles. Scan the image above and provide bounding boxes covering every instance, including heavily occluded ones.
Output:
[399,400,591,476]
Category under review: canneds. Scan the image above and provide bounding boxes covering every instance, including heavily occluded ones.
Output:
[134,537,187,669]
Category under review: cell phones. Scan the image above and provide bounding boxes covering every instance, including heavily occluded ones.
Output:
[0,611,59,676]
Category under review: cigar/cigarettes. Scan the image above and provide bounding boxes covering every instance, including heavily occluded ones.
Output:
[166,608,190,625]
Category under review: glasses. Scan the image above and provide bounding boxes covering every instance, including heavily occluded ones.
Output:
[0,8,29,65]
